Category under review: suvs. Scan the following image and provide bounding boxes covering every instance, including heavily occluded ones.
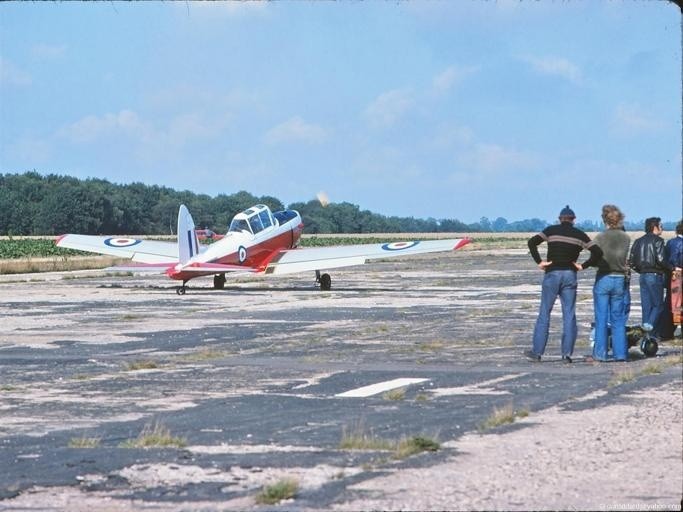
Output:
[194,229,224,240]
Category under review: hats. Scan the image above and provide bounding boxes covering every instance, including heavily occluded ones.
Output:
[560,208,575,217]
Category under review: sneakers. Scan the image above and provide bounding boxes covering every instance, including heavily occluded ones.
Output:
[563,355,571,362]
[524,350,542,360]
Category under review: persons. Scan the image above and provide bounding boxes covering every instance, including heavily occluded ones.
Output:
[522,205,603,363]
[586,205,630,363]
[666,221,683,335]
[628,217,672,340]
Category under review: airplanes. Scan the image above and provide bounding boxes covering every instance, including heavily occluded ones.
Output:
[54,204,474,295]
